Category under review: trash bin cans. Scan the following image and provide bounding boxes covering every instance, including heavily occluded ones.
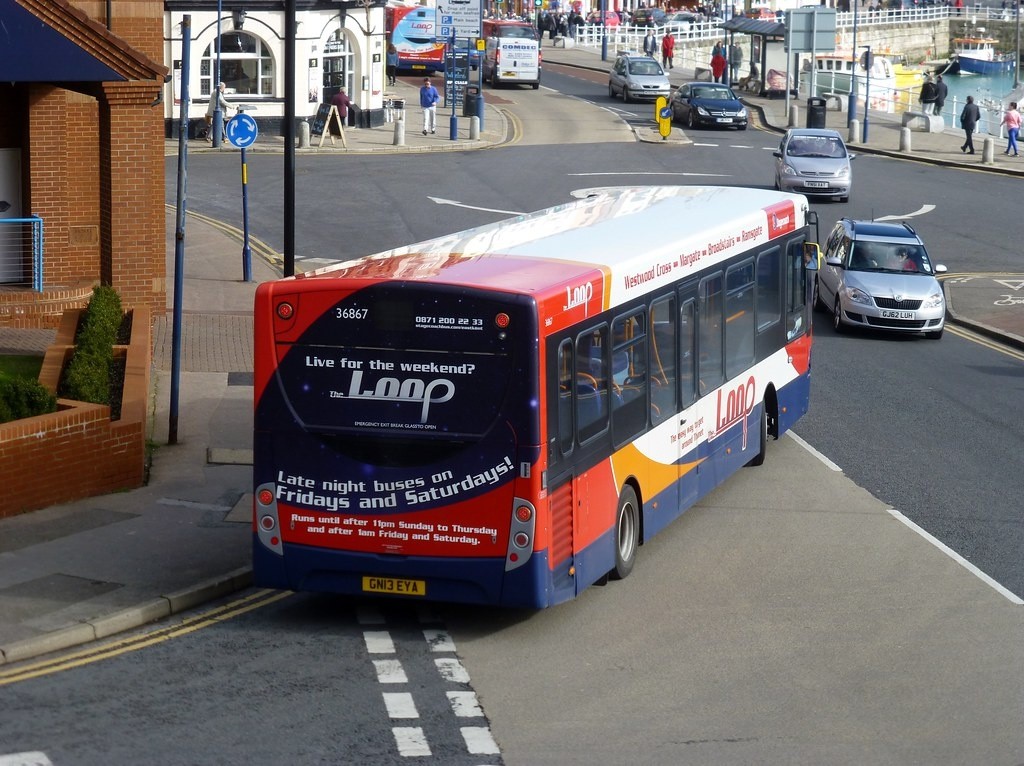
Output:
[463,84,479,116]
[807,97,827,129]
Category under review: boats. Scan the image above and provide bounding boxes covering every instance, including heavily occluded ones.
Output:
[989,1,1023,141]
[949,28,1016,74]
[800,49,934,97]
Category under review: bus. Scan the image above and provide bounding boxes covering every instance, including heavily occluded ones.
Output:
[484,20,544,90]
[251,185,821,623]
[385,5,446,77]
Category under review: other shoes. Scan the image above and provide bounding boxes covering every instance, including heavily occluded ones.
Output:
[967,150,974,154]
[432,131,435,134]
[961,146,966,152]
[1013,153,1019,157]
[669,66,673,68]
[422,130,427,135]
[1004,152,1009,155]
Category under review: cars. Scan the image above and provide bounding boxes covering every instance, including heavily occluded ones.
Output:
[445,40,479,71]
[773,128,856,201]
[668,82,750,131]
[743,8,782,22]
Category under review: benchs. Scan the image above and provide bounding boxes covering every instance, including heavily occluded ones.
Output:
[902,111,945,134]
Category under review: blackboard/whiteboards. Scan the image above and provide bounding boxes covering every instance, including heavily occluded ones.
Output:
[311,104,347,138]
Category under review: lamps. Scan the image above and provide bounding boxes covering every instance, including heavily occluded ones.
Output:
[235,6,248,30]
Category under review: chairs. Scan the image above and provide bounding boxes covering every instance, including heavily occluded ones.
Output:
[576,371,602,431]
[559,385,567,441]
[624,373,662,394]
[650,403,661,420]
[596,378,625,416]
[621,385,641,403]
[789,140,808,154]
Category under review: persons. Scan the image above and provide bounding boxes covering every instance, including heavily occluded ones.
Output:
[332,87,350,127]
[421,79,440,135]
[918,74,948,115]
[1000,101,1022,157]
[644,30,657,57]
[669,3,716,16]
[663,31,674,69]
[710,42,744,83]
[483,8,535,26]
[960,95,980,154]
[744,62,759,91]
[624,2,666,28]
[387,44,399,86]
[945,0,962,16]
[793,140,835,153]
[868,2,883,16]
[888,248,916,270]
[1002,0,1017,19]
[207,82,233,141]
[538,9,603,39]
[806,252,817,268]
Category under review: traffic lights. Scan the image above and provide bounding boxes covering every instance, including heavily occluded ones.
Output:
[495,0,504,3]
[534,0,542,6]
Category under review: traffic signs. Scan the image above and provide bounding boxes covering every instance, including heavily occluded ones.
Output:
[434,0,482,38]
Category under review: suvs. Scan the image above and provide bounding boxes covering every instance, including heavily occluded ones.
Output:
[587,8,708,29]
[606,54,672,102]
[821,217,948,339]
